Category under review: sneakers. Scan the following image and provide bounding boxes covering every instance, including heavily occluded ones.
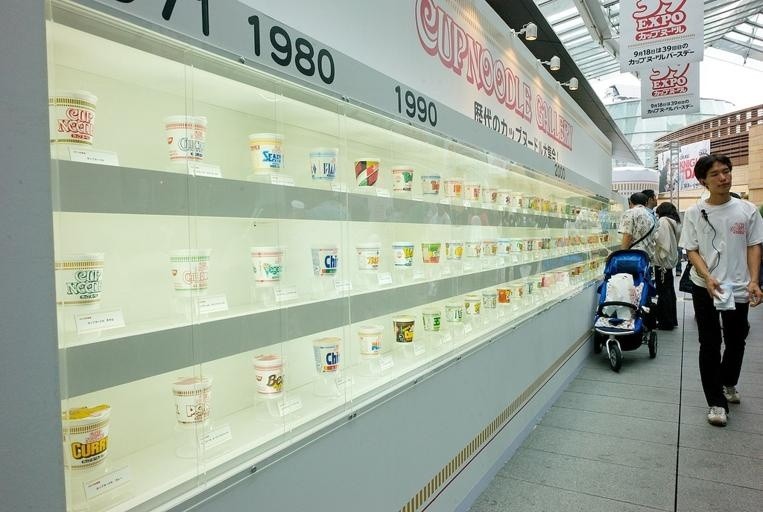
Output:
[656,320,679,331]
[722,385,741,405]
[706,405,728,427]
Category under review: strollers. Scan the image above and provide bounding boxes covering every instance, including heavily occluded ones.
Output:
[591,248,663,376]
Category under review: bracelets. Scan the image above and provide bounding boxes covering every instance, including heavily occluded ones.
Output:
[750,279,759,285]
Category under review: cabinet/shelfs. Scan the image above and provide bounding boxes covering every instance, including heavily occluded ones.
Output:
[39,0,638,510]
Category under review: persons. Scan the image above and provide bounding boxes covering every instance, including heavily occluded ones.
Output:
[618,185,683,332]
[678,150,763,429]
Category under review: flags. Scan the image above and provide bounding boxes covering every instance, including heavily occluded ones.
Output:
[638,62,700,119]
[618,1,704,74]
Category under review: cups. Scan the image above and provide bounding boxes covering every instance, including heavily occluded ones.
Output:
[353,156,380,190]
[163,115,209,175]
[356,242,382,275]
[464,182,619,220]
[441,298,462,322]
[173,375,214,426]
[392,313,415,344]
[310,246,338,279]
[46,89,99,162]
[444,178,462,197]
[419,305,442,333]
[420,173,440,199]
[165,247,214,296]
[57,402,114,471]
[392,241,414,268]
[310,335,342,376]
[358,322,383,355]
[445,241,464,260]
[252,354,286,399]
[392,164,414,194]
[54,252,104,306]
[249,246,285,286]
[421,242,443,265]
[464,255,611,315]
[305,146,338,182]
[466,231,610,259]
[247,133,288,179]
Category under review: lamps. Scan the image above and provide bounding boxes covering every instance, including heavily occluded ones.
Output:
[505,21,578,93]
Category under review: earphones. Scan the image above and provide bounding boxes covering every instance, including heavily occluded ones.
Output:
[703,181,706,186]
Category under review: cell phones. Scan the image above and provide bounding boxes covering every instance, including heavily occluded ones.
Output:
[751,294,756,302]
